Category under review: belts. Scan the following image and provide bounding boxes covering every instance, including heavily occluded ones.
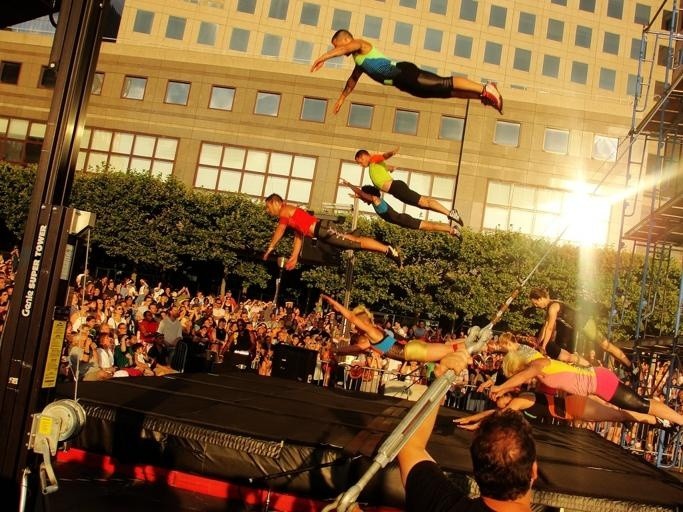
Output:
[312,219,322,248]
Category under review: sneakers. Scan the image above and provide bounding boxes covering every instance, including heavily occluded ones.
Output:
[386,243,403,270]
[450,226,464,243]
[480,82,503,115]
[655,417,674,436]
[447,209,463,226]
[630,362,642,380]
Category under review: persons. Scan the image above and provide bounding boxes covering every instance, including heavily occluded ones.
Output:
[396,350,539,512]
[262,192,403,273]
[1,243,681,472]
[354,144,463,225]
[336,175,462,242]
[309,29,504,117]
